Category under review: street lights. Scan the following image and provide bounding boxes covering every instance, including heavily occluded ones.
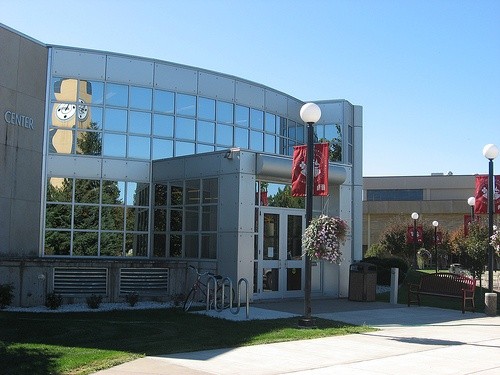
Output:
[482,144,494,293]
[467,197,476,286]
[412,211,419,271]
[432,221,438,274]
[300,103,322,325]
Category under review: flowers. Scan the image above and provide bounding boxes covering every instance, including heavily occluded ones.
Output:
[299,213,355,267]
[489,225,500,257]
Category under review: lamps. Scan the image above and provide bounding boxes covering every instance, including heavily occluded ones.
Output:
[223,147,241,159]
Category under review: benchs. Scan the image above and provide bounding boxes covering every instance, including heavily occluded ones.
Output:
[407,272,476,314]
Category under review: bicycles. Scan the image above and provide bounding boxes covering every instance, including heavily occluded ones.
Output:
[182,265,238,313]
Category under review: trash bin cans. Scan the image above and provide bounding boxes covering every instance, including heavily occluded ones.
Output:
[349,263,378,302]
